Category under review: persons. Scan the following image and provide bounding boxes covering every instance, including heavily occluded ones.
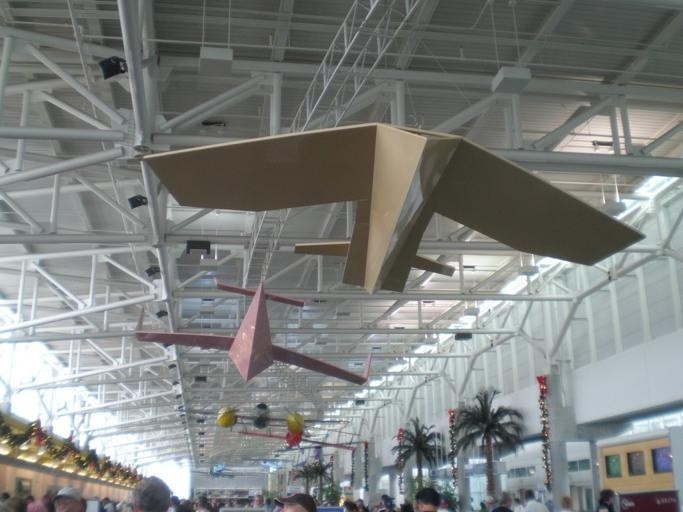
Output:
[1,476,615,512]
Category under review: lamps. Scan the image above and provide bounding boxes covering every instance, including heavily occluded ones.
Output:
[144,266,160,278]
[96,55,127,80]
[127,193,147,210]
[155,310,167,318]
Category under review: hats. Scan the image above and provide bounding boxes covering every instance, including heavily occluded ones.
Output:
[53,487,84,502]
[273,493,316,511]
